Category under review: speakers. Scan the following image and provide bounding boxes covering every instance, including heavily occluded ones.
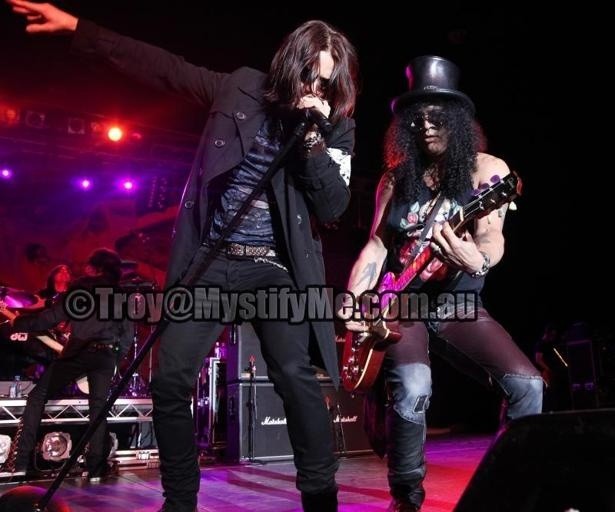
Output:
[453,407,615,512]
[319,381,387,458]
[224,383,294,464]
[220,321,269,381]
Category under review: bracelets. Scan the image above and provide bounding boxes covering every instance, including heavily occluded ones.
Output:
[470,252,493,281]
[300,133,323,148]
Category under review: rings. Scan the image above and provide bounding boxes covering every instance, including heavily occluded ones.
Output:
[433,247,446,262]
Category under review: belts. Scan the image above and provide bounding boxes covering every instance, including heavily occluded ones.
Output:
[203,238,275,260]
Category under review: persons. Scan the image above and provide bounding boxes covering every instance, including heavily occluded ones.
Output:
[5,247,141,478]
[38,262,73,302]
[337,57,548,512]
[1,1,361,512]
[531,322,561,373]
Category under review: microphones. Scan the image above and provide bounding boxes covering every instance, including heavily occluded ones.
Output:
[302,108,333,133]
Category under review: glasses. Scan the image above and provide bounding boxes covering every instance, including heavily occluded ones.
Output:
[299,65,334,90]
[406,111,451,129]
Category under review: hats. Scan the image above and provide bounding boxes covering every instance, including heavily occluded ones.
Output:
[391,56,476,117]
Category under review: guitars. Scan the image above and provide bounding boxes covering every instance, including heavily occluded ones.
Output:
[339,171,523,399]
[0,298,116,396]
